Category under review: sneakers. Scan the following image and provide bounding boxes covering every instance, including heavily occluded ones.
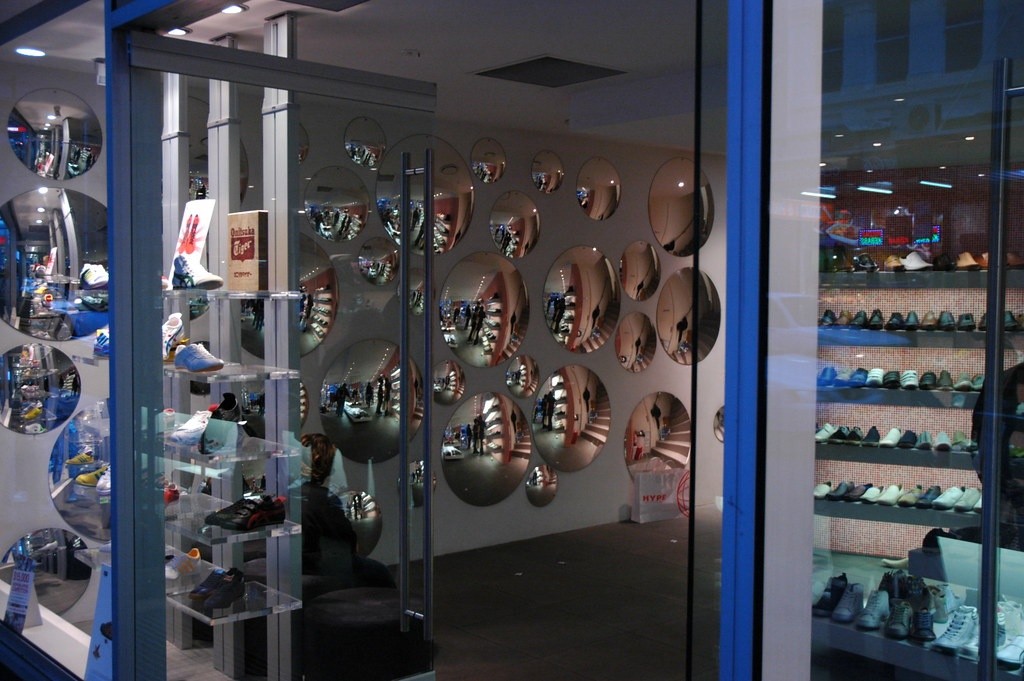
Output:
[190,297,210,322]
[170,410,213,445]
[481,293,501,351]
[162,313,185,360]
[433,221,449,253]
[174,343,224,372]
[812,247,1024,664]
[559,284,576,343]
[162,276,168,289]
[198,392,242,455]
[189,567,249,610]
[484,404,502,451]
[204,494,286,530]
[165,548,201,580]
[14,262,110,553]
[310,286,333,338]
[172,255,224,289]
[554,381,567,430]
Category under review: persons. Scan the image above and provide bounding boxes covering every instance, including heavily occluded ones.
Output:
[533,391,555,431]
[329,384,359,417]
[367,376,391,416]
[464,302,486,346]
[467,415,485,456]
[410,207,421,231]
[301,434,439,660]
[500,227,511,255]
[553,297,566,335]
[315,210,352,239]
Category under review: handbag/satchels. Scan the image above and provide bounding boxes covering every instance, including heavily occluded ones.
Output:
[631,456,690,523]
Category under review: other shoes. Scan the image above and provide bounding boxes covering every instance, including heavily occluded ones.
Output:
[376,410,382,414]
[164,482,179,507]
[385,409,389,416]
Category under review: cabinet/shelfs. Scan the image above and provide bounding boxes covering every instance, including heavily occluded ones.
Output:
[73,283,307,625]
[811,269,1024,681]
